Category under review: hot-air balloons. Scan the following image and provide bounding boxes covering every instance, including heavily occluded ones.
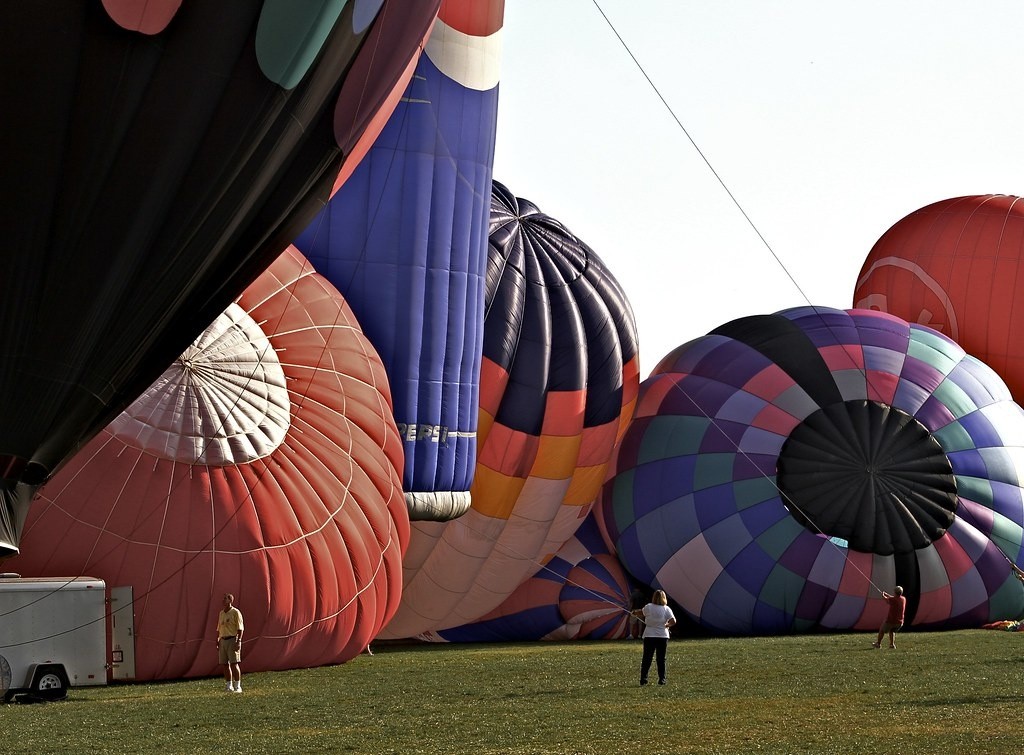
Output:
[594,305,1024,634]
[1,239,412,676]
[851,195,1024,415]
[367,180,640,637]
[294,0,504,518]
[435,512,643,642]
[1,0,438,465]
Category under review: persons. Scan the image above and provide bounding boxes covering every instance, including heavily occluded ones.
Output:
[631,590,676,685]
[872,586,906,649]
[216,592,244,693]
[367,643,374,656]
[1012,563,1024,582]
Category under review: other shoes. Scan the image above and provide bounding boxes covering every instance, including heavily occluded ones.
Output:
[872,642,881,649]
[234,687,242,693]
[226,686,234,692]
[658,681,666,685]
[889,645,896,649]
[640,680,648,685]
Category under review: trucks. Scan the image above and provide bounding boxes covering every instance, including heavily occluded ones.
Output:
[0,573,135,704]
